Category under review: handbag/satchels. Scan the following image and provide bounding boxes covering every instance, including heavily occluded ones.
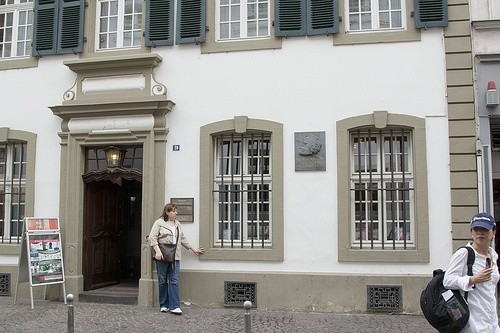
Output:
[420,246,475,333]
[152,242,177,262]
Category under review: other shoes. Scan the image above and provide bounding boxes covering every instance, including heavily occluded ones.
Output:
[161,307,169,312]
[170,307,182,313]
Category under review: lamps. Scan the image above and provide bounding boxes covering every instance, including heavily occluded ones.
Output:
[104,145,125,177]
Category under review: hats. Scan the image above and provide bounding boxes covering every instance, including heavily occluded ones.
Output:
[470,213,496,230]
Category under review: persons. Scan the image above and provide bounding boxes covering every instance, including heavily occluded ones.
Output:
[148,202,205,313]
[443,211,500,333]
